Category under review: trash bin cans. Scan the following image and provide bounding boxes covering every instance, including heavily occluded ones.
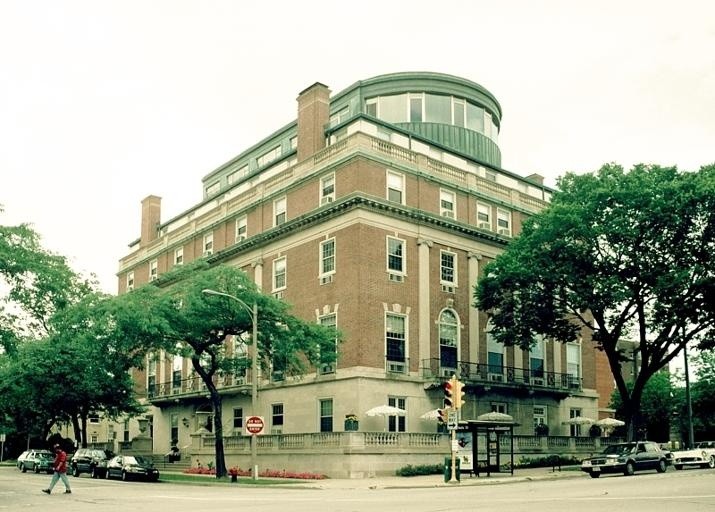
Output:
[445,456,460,483]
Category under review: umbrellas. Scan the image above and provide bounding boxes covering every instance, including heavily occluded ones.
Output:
[562,415,594,437]
[364,405,406,432]
[478,410,513,421]
[420,408,444,420]
[595,417,625,438]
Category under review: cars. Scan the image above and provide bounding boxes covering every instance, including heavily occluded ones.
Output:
[106,455,161,480]
[670,441,715,469]
[580,441,671,479]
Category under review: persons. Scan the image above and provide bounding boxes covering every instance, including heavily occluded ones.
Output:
[41,443,71,495]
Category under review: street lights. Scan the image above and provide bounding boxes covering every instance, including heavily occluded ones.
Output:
[202,288,260,482]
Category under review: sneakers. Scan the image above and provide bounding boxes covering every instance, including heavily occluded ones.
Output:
[42,489,50,494]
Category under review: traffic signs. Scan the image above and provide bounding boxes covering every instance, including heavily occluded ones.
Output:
[447,410,458,431]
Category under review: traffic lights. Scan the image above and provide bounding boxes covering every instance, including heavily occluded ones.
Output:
[443,381,454,406]
[437,409,447,425]
[459,382,467,406]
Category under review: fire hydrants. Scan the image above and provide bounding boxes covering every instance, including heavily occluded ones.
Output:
[230,467,238,482]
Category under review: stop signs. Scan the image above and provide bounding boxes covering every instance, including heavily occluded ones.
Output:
[246,415,264,436]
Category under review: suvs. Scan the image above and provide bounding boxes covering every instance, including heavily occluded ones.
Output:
[70,448,116,477]
[17,449,56,474]
